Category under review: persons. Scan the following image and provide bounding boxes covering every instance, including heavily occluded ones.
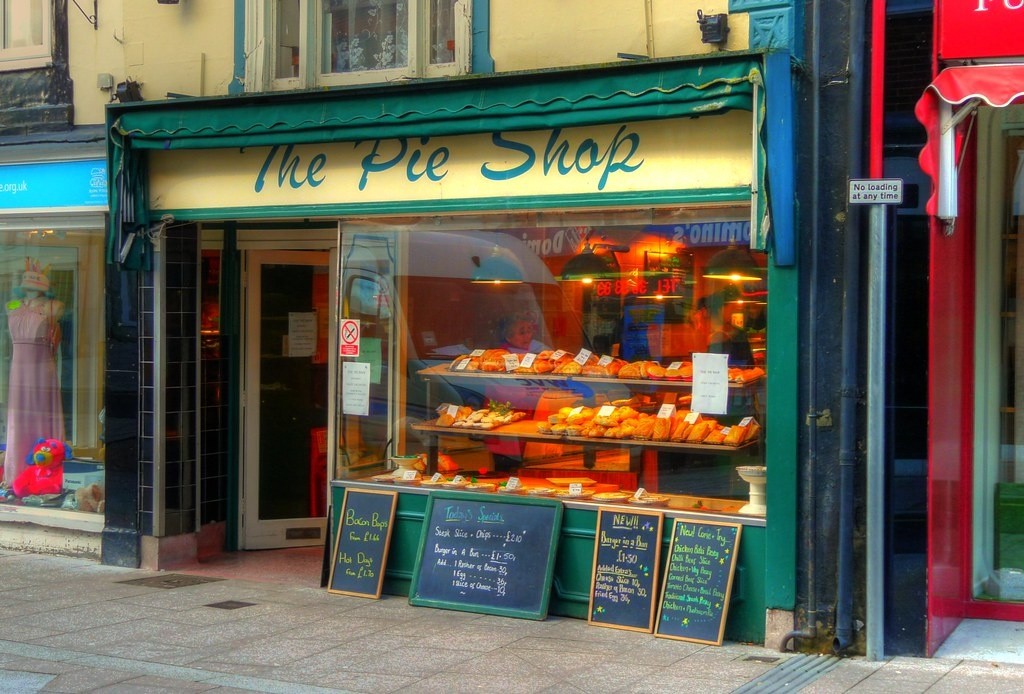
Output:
[690,296,760,455]
[485,313,555,474]
[2,257,65,488]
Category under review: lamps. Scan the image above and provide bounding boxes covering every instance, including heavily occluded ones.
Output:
[635,232,683,298]
[469,230,524,284]
[553,228,615,282]
[701,235,762,281]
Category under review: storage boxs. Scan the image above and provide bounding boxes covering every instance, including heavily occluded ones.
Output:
[61,460,105,491]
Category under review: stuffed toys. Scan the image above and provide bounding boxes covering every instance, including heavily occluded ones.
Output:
[11,438,105,513]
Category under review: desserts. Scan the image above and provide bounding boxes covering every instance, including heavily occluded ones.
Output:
[373,473,669,505]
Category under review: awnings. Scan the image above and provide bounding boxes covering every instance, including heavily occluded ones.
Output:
[107,52,1024,261]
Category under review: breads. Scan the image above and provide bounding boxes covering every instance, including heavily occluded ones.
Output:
[436,348,763,444]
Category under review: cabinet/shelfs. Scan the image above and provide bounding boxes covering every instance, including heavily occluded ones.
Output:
[410,362,765,500]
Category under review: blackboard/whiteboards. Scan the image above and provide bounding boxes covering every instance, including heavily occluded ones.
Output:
[326,487,398,600]
[407,490,564,621]
[653,517,743,646]
[587,505,665,633]
